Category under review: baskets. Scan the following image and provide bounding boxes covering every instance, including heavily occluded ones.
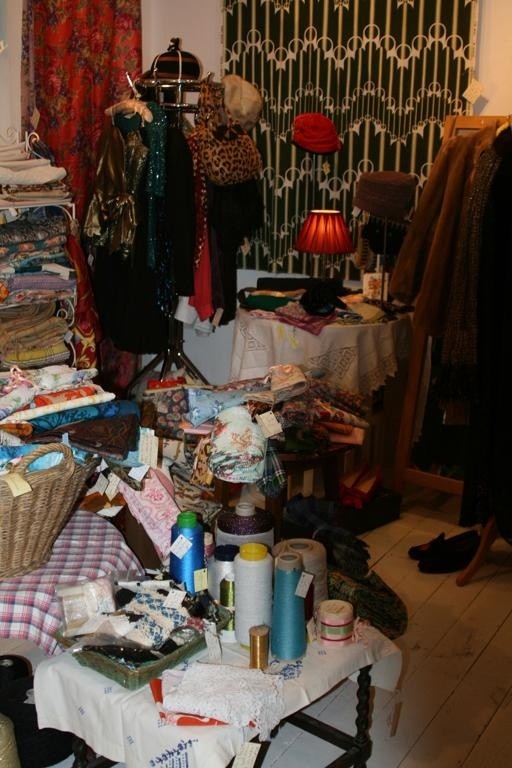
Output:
[54,605,233,690]
[0,445,98,578]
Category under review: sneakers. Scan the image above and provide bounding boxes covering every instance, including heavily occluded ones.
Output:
[408,528,480,574]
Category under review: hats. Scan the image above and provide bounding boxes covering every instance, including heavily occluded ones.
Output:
[291,112,341,153]
[138,50,200,79]
[353,172,417,222]
[222,74,263,130]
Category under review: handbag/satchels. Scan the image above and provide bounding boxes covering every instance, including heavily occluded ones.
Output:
[193,121,260,187]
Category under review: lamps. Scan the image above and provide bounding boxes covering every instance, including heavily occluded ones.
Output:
[287,208,357,283]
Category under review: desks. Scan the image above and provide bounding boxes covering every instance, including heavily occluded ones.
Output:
[229,297,410,470]
